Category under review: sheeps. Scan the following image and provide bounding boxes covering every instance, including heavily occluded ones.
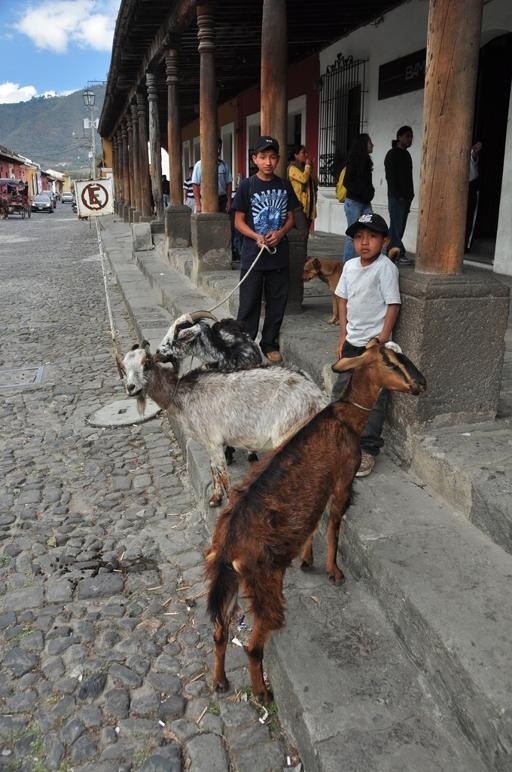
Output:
[115,336,332,507]
[152,308,262,466]
[201,336,428,705]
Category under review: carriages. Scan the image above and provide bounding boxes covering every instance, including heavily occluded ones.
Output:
[0,177,31,219]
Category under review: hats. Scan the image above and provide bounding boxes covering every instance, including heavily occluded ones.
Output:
[253,135,278,153]
[346,213,388,238]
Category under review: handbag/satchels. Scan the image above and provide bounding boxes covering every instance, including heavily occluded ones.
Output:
[336,166,348,203]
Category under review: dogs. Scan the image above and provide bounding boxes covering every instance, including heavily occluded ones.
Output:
[303,247,401,326]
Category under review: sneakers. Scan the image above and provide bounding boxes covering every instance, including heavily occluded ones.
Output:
[265,351,282,363]
[354,451,376,477]
[395,256,412,265]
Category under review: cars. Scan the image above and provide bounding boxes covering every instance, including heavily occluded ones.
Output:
[32,191,59,214]
[61,192,73,202]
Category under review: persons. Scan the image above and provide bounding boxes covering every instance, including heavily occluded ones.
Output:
[382,124,417,260]
[329,213,400,482]
[160,174,170,208]
[287,144,320,236]
[183,167,197,213]
[341,133,377,266]
[234,136,302,363]
[466,140,488,249]
[191,132,232,214]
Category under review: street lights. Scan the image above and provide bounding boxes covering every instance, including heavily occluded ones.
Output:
[82,87,100,181]
